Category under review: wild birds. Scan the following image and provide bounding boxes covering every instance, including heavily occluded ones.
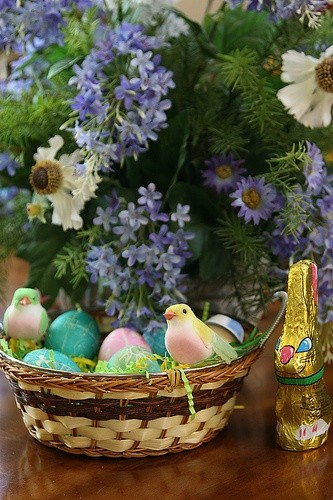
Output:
[162,303,238,365]
[3,287,49,351]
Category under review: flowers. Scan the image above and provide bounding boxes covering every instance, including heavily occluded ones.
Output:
[0,0,333,345]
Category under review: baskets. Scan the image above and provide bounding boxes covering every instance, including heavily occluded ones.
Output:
[1,289,288,458]
